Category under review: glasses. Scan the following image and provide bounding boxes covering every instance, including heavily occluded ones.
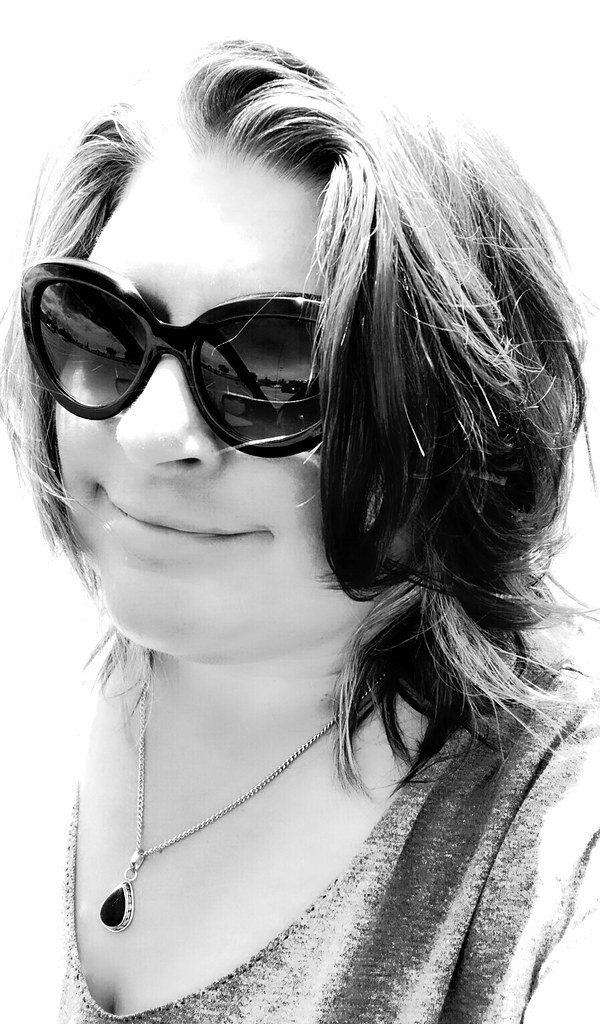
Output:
[21,256,328,458]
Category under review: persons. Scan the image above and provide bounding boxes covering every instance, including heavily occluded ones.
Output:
[1,35,600,1024]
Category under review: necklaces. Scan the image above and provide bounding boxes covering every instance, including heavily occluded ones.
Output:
[96,648,386,933]
